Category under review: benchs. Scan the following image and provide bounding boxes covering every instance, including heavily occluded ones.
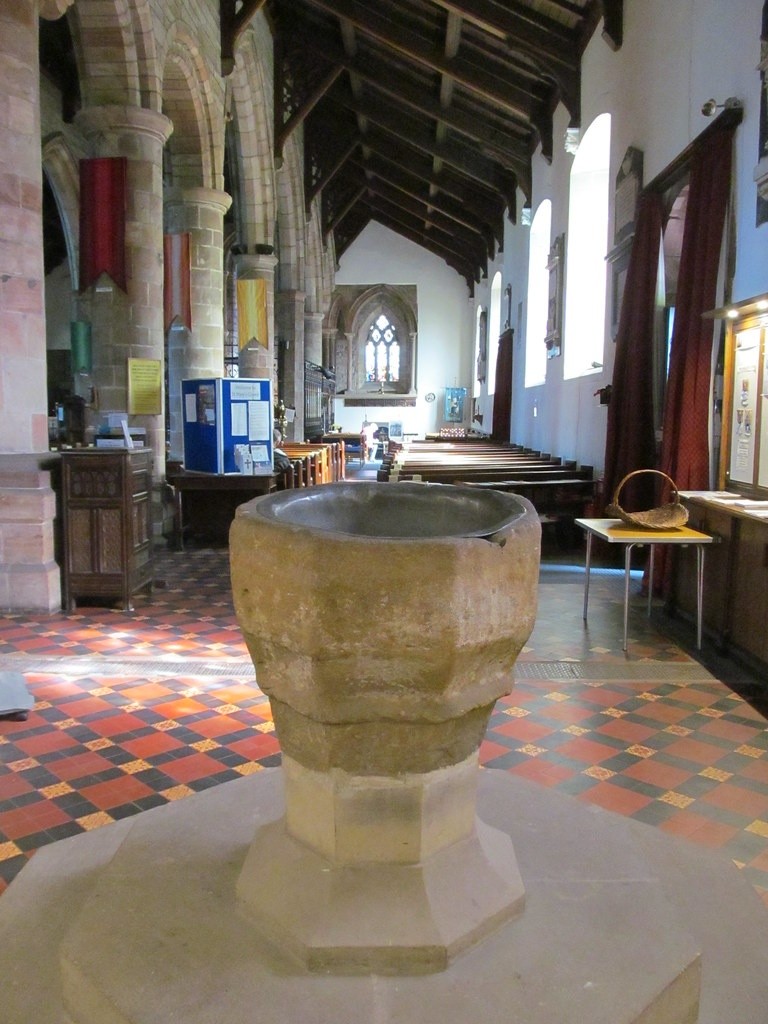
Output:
[377,439,598,551]
[276,433,367,501]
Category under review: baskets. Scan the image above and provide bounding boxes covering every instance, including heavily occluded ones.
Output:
[605,470,689,528]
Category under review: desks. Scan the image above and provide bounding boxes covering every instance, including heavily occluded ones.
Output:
[575,518,723,652]
[175,473,278,549]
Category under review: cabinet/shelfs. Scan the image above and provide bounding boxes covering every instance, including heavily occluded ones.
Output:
[60,447,155,612]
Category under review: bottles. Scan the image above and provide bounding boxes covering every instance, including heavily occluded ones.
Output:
[439,428,466,438]
[306,438,310,445]
